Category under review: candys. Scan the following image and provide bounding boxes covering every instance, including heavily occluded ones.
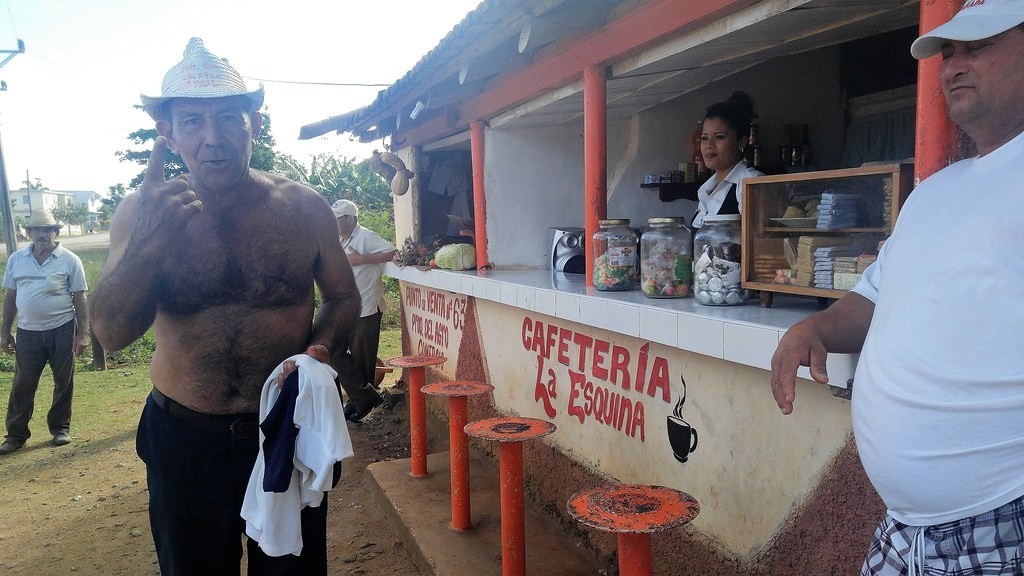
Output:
[593,255,690,297]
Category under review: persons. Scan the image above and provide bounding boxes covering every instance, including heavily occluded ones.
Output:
[0,208,91,455]
[690,91,766,294]
[88,36,361,576]
[771,0,1024,576]
[330,199,400,422]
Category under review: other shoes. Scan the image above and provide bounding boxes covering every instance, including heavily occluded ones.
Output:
[0,439,24,455]
[53,434,71,445]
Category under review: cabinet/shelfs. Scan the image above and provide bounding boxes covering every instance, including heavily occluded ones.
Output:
[738,158,916,302]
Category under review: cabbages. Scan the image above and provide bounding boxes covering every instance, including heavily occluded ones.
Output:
[433,243,477,270]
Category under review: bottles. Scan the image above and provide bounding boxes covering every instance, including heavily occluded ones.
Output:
[694,121,707,175]
[694,215,749,306]
[744,123,760,167]
[591,219,637,292]
[640,217,693,299]
[800,123,811,166]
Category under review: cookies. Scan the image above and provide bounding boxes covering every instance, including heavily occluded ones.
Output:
[752,254,789,284]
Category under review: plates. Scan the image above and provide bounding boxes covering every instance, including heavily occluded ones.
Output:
[770,219,816,227]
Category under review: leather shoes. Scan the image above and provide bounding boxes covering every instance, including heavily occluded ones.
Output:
[343,404,355,419]
[350,394,385,421]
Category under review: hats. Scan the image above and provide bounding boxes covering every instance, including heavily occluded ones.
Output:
[139,38,264,121]
[21,208,64,230]
[331,199,358,218]
[911,0,1024,59]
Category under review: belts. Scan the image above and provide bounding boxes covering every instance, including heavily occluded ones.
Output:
[152,386,259,439]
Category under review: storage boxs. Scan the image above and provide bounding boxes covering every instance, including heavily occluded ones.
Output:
[642,162,696,184]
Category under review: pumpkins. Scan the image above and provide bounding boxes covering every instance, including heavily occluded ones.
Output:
[371,150,410,196]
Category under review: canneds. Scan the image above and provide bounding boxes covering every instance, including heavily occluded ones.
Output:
[791,143,801,166]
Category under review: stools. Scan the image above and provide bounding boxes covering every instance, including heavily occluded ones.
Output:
[458,416,559,576]
[565,483,701,576]
[385,353,446,480]
[419,379,496,534]
